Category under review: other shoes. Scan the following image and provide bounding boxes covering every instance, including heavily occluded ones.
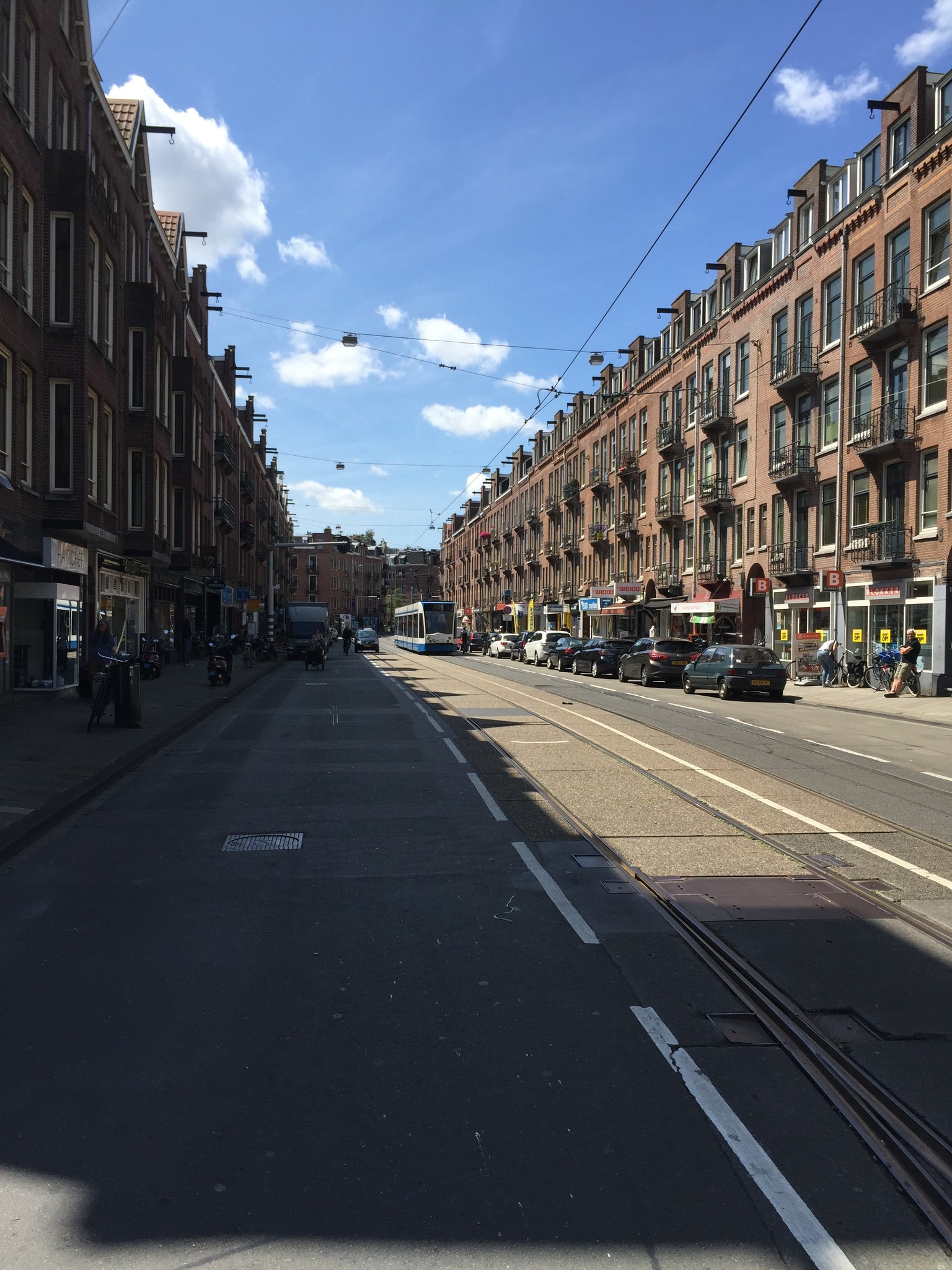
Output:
[822,684,830,687]
[825,681,834,687]
[894,696,899,698]
[884,693,896,698]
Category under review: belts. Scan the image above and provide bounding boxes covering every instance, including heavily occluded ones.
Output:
[818,649,829,652]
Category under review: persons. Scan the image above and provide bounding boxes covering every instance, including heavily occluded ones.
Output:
[312,629,326,644]
[85,618,117,708]
[213,621,226,641]
[180,615,194,666]
[560,624,570,634]
[460,627,469,653]
[342,626,353,653]
[816,640,839,688]
[883,628,921,698]
[498,626,504,633]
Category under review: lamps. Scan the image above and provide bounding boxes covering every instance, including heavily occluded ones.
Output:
[589,353,604,366]
[336,525,341,529]
[341,334,357,347]
[336,462,345,470]
[482,466,490,474]
[406,562,410,566]
[430,526,435,530]
[359,565,362,568]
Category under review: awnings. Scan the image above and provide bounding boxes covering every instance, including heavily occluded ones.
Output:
[456,594,740,614]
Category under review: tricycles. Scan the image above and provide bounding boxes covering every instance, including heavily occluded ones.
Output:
[304,636,328,671]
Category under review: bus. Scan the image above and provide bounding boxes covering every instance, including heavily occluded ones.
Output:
[395,600,458,656]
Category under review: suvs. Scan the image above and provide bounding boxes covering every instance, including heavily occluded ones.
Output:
[328,627,339,642]
[510,632,535,662]
[522,630,573,666]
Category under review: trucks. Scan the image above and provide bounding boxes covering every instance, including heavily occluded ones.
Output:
[284,601,332,660]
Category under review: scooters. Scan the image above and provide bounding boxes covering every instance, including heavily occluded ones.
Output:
[134,629,169,680]
[206,634,237,685]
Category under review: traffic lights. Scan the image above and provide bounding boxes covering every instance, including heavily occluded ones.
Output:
[376,593,381,601]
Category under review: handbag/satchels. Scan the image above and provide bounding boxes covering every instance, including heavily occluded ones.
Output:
[847,660,862,674]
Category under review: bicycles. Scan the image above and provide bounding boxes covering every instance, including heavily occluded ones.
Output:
[829,643,868,688]
[83,651,133,733]
[342,638,353,656]
[240,634,279,669]
[863,645,923,698]
[191,630,210,661]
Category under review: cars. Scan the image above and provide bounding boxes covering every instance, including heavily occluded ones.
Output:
[489,632,520,658]
[682,644,787,701]
[456,626,499,656]
[617,637,701,689]
[352,628,381,653]
[571,638,637,679]
[547,637,593,671]
